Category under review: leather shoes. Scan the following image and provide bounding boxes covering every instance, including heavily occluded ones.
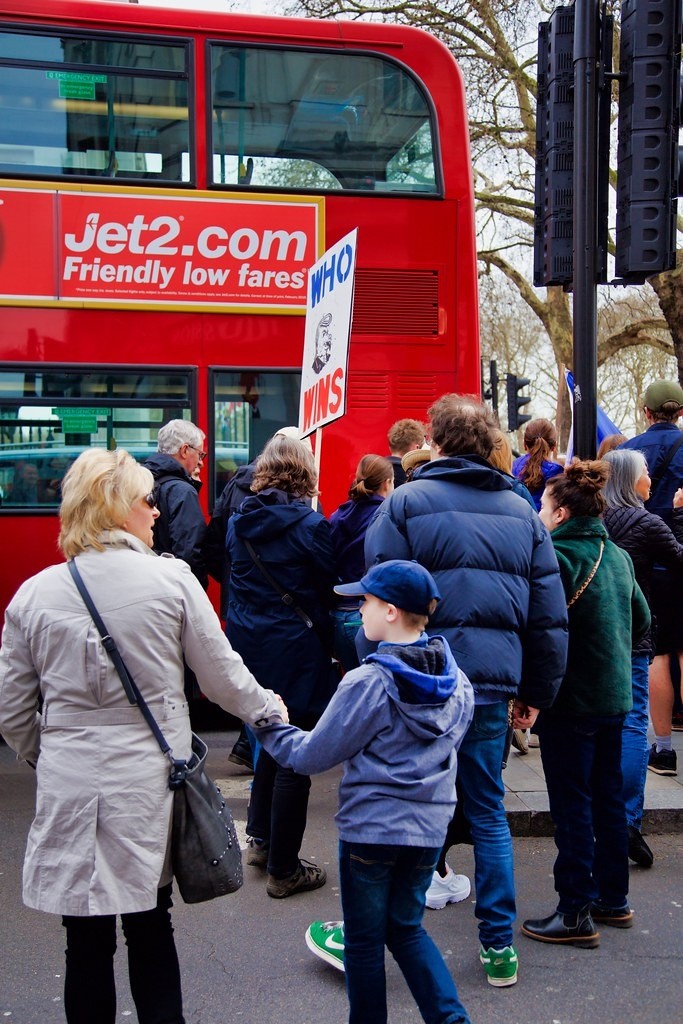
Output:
[587,901,632,929]
[520,909,599,948]
[226,738,254,770]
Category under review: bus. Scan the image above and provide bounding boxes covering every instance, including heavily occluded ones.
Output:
[0,1,483,645]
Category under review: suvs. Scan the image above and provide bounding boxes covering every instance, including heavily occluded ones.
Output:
[1,440,248,508]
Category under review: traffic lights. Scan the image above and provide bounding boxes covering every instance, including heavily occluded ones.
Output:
[507,374,532,431]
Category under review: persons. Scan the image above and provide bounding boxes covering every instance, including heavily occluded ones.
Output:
[1,449,288,1024]
[597,381,683,870]
[223,437,334,899]
[303,394,569,987]
[513,419,564,512]
[522,457,651,953]
[252,560,473,1024]
[387,419,426,488]
[205,427,313,768]
[329,454,394,676]
[486,428,537,513]
[140,419,209,591]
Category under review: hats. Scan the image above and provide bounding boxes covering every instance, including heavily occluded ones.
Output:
[400,448,430,473]
[644,379,683,413]
[333,560,440,614]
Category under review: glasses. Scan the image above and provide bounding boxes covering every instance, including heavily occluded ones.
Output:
[143,494,156,509]
[180,444,205,460]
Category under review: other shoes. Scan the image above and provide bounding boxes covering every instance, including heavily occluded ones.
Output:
[528,733,540,746]
[513,728,528,756]
[625,826,653,869]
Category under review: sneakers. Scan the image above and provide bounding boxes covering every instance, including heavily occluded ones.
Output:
[648,743,677,775]
[305,920,345,971]
[245,836,269,866]
[477,943,517,986]
[266,858,326,899]
[425,862,470,910]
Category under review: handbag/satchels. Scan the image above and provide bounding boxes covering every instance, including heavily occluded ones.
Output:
[169,731,243,903]
